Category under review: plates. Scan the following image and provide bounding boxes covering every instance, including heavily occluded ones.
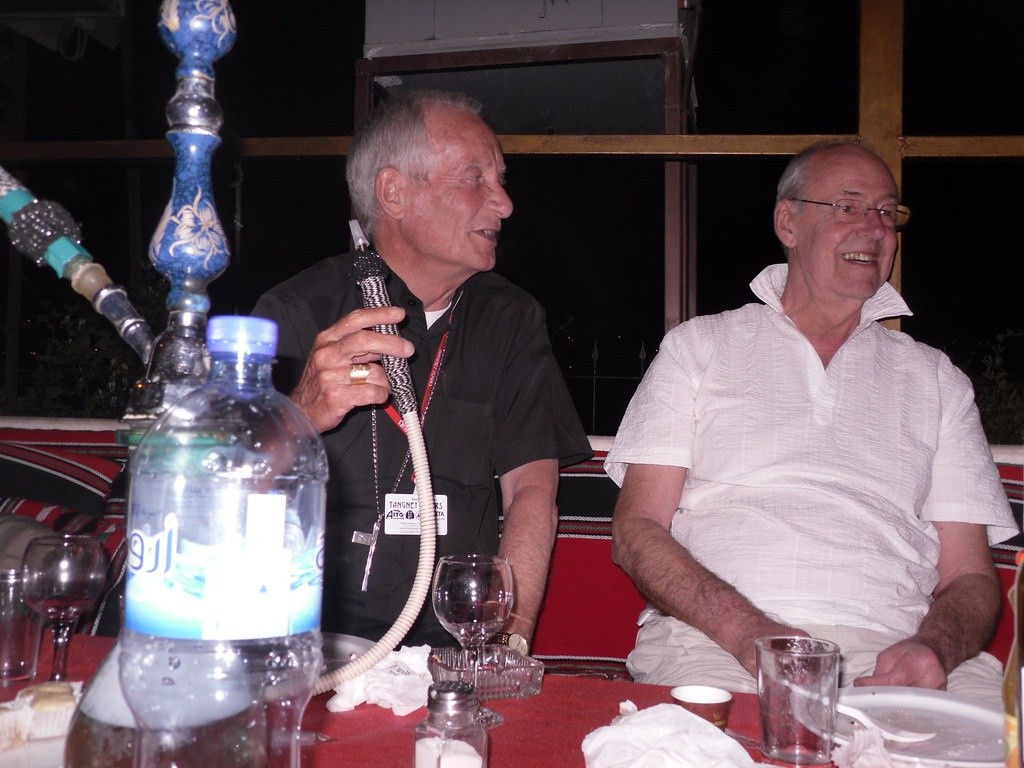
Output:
[791,686,1005,767]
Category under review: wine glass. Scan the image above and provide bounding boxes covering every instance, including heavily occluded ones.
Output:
[432,555,514,729]
[22,535,107,680]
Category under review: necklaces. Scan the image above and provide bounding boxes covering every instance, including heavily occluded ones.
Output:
[352,288,463,592]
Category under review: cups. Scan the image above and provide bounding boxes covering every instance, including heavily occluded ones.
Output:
[671,685,734,731]
[753,635,841,764]
[0,569,42,689]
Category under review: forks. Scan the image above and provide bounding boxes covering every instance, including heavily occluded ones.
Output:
[783,680,937,742]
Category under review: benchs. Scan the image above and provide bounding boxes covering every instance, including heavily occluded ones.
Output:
[3,426,1024,674]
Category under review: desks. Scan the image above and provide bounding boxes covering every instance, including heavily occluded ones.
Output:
[1,627,844,767]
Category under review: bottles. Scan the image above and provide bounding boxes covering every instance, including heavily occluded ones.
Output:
[120,316,331,768]
[412,680,488,768]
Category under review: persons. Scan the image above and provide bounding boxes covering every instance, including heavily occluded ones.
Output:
[602,141,1020,689]
[252,91,595,663]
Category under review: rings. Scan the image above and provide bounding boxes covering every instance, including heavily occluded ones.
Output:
[351,363,371,384]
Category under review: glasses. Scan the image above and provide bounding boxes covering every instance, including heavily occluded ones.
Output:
[792,198,912,230]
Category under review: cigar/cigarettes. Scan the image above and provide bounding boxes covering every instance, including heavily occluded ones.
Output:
[431,653,447,664]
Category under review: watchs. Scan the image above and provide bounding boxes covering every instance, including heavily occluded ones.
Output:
[486,631,529,656]
